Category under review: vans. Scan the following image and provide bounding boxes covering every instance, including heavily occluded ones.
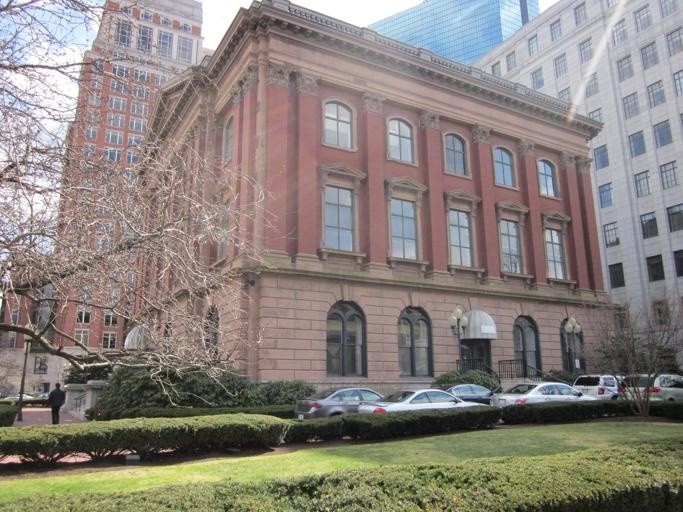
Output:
[572,374,683,405]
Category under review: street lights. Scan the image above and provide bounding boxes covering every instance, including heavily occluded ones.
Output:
[450,308,468,374]
[563,317,580,375]
[16,321,34,421]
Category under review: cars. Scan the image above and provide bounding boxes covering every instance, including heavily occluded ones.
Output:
[2,391,50,406]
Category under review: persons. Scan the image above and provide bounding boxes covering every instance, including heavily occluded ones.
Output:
[49,383,65,425]
